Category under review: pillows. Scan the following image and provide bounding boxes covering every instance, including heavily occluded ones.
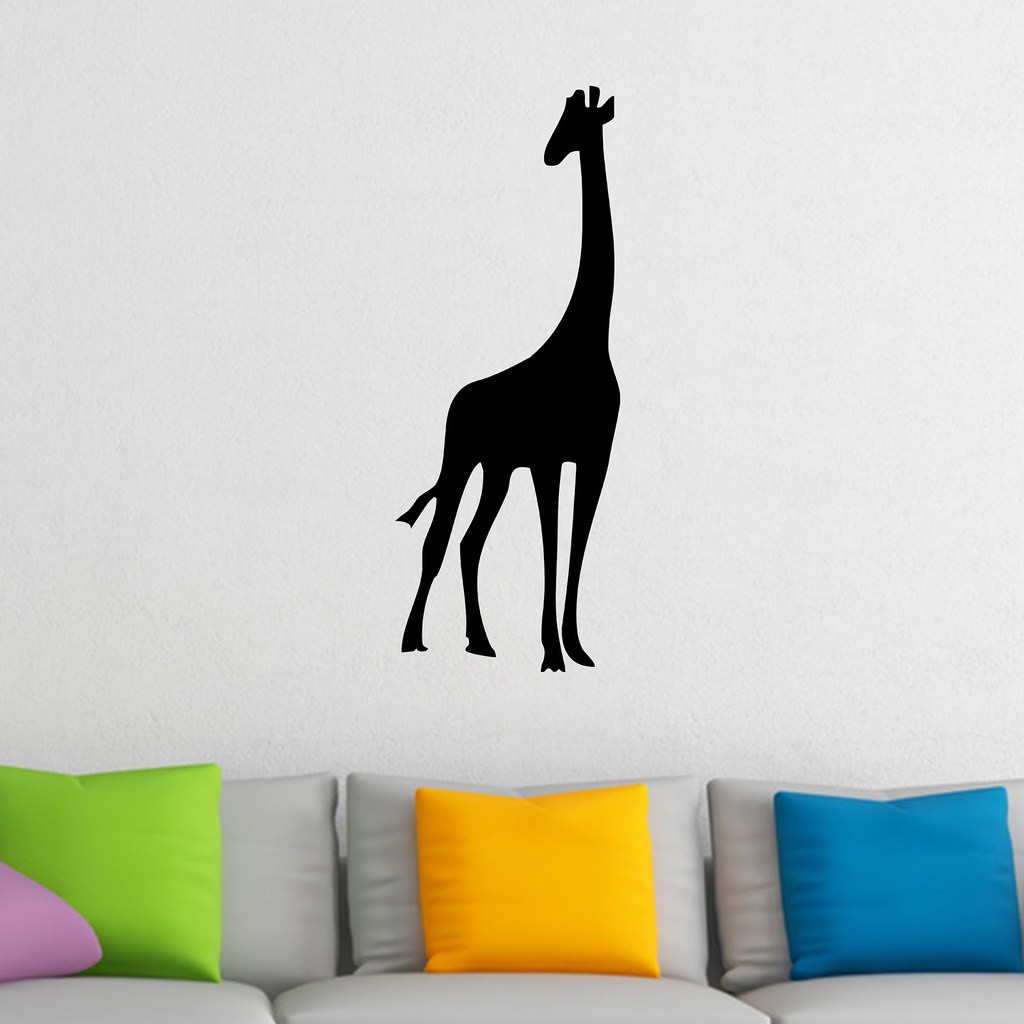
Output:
[0,766,225,984]
[774,786,1024,984]
[415,786,662,978]
[223,771,340,1001]
[1,863,102,982]
[348,774,712,979]
[709,779,1024,993]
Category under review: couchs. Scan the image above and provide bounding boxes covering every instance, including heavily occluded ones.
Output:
[1,764,1024,1024]
